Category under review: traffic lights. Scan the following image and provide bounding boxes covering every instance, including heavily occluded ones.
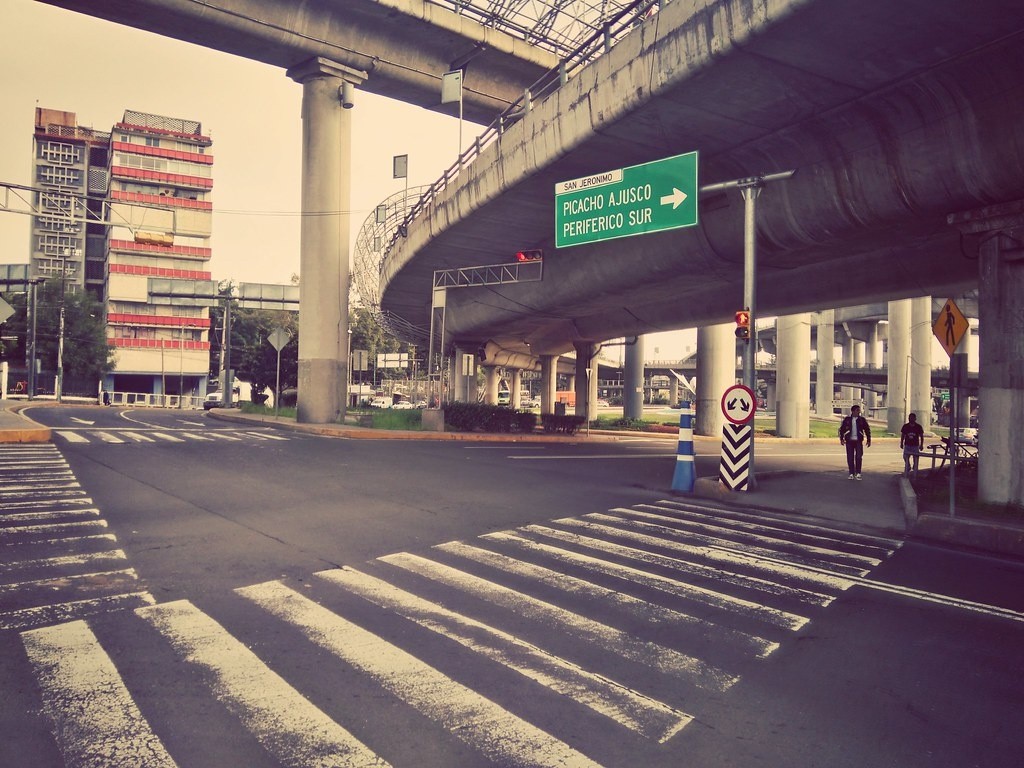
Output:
[735,311,751,340]
[514,248,543,263]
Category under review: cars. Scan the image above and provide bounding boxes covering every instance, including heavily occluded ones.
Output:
[530,396,541,407]
[371,397,428,410]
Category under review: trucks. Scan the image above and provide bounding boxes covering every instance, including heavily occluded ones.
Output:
[203,382,274,409]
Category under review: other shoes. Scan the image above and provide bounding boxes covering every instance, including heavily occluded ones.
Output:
[848,474,854,480]
[855,472,862,480]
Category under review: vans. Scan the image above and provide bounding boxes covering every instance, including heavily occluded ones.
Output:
[15,381,47,394]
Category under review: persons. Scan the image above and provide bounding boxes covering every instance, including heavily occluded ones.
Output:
[103,390,110,405]
[900,413,924,478]
[839,405,871,481]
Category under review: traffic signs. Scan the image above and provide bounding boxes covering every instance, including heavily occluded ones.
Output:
[553,151,700,248]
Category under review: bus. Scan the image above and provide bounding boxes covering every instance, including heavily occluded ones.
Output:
[498,390,531,405]
